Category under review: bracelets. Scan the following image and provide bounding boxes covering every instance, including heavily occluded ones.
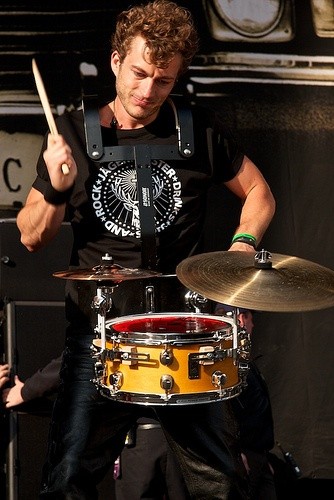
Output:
[41,184,72,205]
[232,235,259,250]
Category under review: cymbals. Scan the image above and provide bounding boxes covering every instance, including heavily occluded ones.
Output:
[175,250,333,313]
[51,263,162,281]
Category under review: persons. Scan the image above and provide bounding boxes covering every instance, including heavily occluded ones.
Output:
[17,1,276,500]
[1,307,275,500]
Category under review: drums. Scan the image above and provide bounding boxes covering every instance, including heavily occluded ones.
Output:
[88,311,252,407]
